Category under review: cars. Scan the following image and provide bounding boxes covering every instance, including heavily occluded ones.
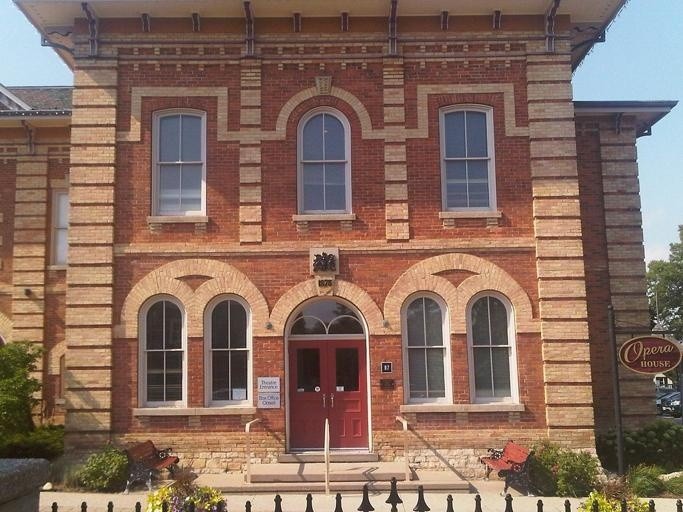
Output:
[656,392,681,417]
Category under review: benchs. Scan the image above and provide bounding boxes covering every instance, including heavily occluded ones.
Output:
[123,439,180,495]
[481,439,535,497]
